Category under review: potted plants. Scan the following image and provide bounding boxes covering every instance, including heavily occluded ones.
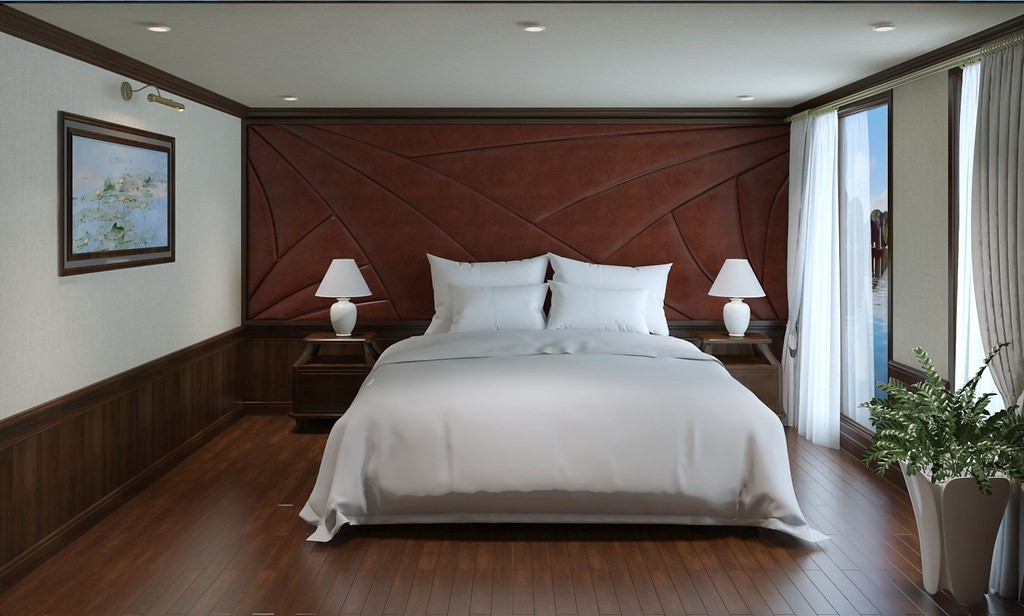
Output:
[856,339,1024,602]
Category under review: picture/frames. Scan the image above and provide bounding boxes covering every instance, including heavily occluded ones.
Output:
[58,109,175,278]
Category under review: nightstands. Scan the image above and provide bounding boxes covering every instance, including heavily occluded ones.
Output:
[695,332,786,419]
[289,331,381,427]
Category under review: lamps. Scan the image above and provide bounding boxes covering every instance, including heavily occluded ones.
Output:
[121,81,184,113]
[314,258,372,337]
[708,259,766,336]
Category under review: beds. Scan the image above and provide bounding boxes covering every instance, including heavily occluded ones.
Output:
[299,334,829,542]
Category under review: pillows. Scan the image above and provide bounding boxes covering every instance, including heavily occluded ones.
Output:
[424,252,673,338]
